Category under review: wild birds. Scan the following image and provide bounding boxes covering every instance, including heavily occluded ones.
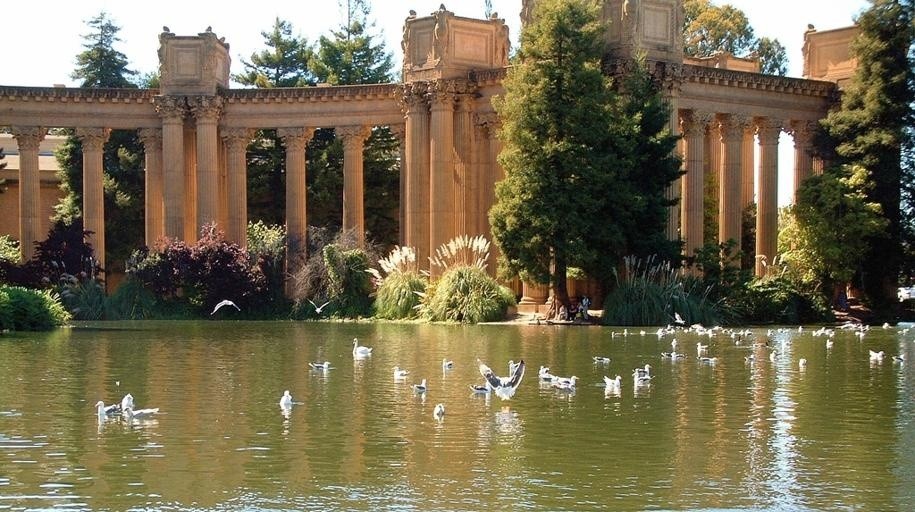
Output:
[766,325,872,339]
[658,338,686,364]
[306,297,331,313]
[96,394,159,421]
[433,403,445,418]
[733,335,746,347]
[883,323,910,335]
[825,339,838,349]
[769,350,782,362]
[602,364,656,387]
[798,356,809,363]
[443,359,453,369]
[280,390,293,408]
[411,378,427,395]
[609,325,756,338]
[353,338,374,357]
[750,341,775,350]
[592,354,612,363]
[868,350,906,363]
[211,300,241,315]
[391,366,410,377]
[674,312,684,324]
[743,354,755,363]
[695,343,722,363]
[470,359,580,401]
[308,361,332,369]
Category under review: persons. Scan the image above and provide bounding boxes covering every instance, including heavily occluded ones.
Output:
[557,294,590,324]
[498,18,512,67]
[402,9,417,64]
[156,25,177,80]
[430,3,455,67]
[801,21,819,77]
[198,25,218,79]
[487,12,505,67]
